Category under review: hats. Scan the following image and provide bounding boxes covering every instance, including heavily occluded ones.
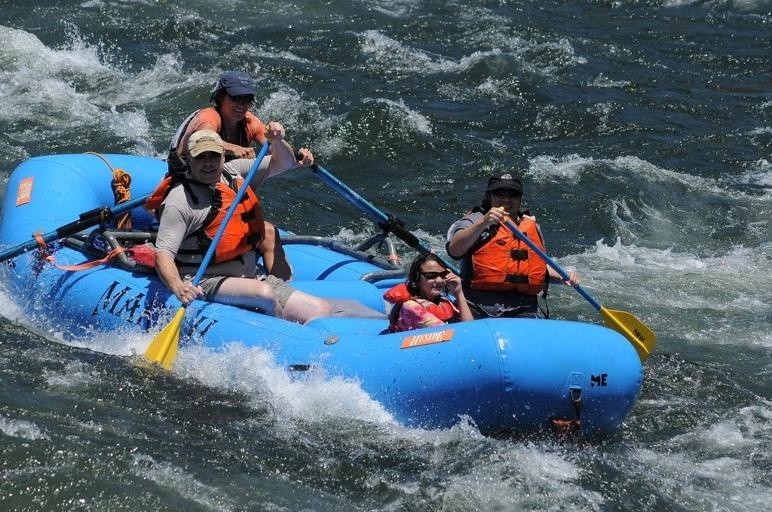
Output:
[187,129,224,158]
[218,70,258,96]
[486,172,523,195]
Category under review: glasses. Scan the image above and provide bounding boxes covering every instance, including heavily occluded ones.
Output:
[225,91,253,102]
[492,187,520,198]
[420,271,448,280]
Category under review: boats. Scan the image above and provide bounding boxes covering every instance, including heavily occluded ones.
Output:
[2,151,641,445]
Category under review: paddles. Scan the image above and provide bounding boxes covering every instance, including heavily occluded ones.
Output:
[145,139,271,374]
[500,215,657,362]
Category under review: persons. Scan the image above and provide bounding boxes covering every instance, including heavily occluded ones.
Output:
[379,253,473,335]
[145,71,313,282]
[154,121,332,326]
[446,173,582,324]
